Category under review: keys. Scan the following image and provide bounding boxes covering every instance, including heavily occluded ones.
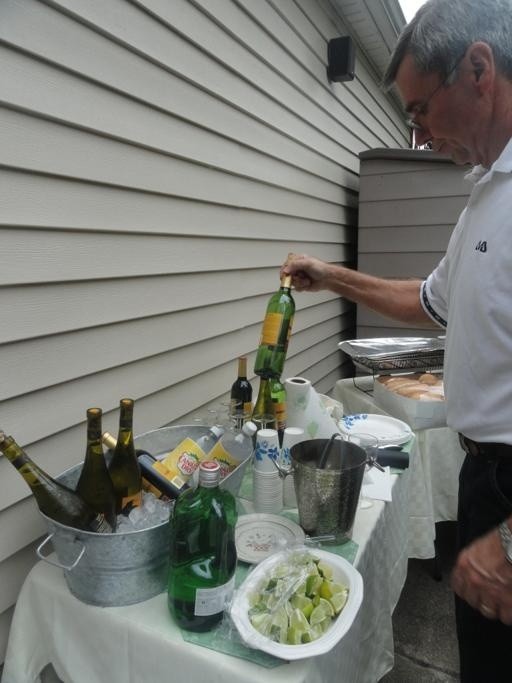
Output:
[365,444,409,471]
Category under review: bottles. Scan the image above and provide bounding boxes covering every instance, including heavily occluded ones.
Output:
[272,378,286,448]
[167,460,236,632]
[160,425,223,485]
[109,398,141,515]
[231,356,252,427]
[101,432,190,499]
[202,420,257,479]
[254,252,299,380]
[75,408,117,527]
[252,377,279,445]
[0,430,113,545]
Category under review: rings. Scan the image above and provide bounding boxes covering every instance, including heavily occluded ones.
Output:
[478,603,498,618]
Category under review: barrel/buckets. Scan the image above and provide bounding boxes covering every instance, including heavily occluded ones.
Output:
[269,433,384,548]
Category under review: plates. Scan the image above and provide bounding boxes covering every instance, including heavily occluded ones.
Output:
[336,413,412,448]
[344,354,444,370]
[233,514,306,564]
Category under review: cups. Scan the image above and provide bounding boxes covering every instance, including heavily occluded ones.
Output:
[219,398,243,432]
[193,413,206,425]
[227,410,250,433]
[347,431,379,510]
[282,427,306,508]
[207,406,225,426]
[253,429,283,515]
[253,414,276,449]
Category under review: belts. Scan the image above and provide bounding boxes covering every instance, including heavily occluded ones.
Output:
[456,435,511,460]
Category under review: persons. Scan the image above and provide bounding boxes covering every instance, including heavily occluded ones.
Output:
[281,2,512,683]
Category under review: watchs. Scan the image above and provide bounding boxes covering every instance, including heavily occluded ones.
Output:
[498,521,512,562]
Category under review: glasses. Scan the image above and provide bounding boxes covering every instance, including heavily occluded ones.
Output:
[406,46,471,134]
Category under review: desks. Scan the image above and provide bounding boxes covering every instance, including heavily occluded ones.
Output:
[4,429,417,683]
[335,369,468,582]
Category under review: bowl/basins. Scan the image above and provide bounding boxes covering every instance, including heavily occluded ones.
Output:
[229,546,364,661]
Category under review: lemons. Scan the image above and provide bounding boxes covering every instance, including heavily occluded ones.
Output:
[249,551,348,645]
